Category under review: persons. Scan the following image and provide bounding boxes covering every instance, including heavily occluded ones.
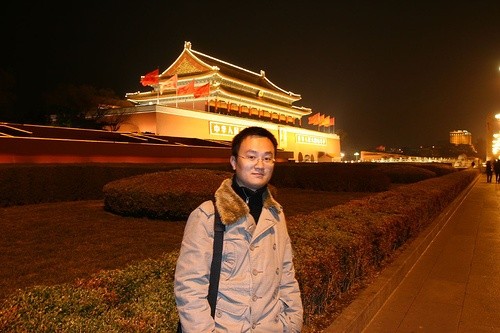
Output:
[470,158,500,184]
[173,126,306,333]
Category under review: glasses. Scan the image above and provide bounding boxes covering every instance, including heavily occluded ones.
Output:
[236,155,276,167]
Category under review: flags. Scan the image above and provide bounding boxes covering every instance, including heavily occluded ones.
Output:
[193,81,211,98]
[161,73,177,89]
[174,81,196,95]
[139,68,161,87]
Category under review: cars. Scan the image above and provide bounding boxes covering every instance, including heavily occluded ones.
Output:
[481,163,494,173]
